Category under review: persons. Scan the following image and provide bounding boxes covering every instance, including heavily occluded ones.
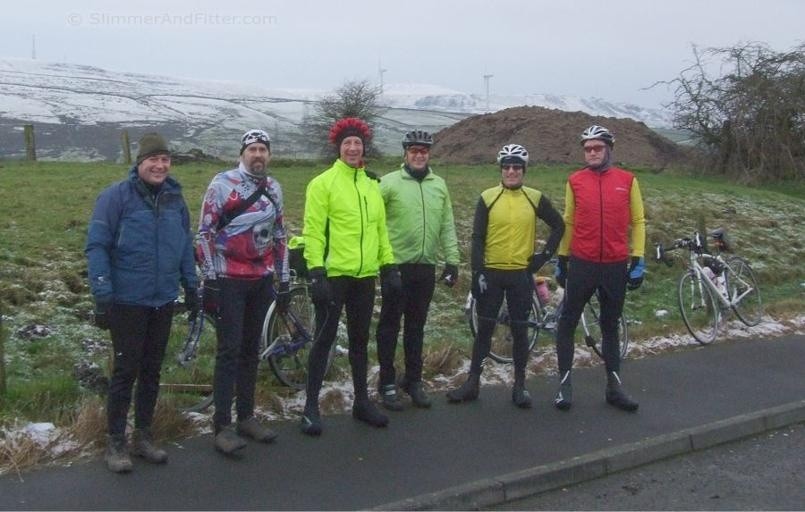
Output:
[445,144,566,409]
[85,132,199,474]
[194,128,290,458]
[300,118,402,436]
[375,129,460,411]
[551,124,646,411]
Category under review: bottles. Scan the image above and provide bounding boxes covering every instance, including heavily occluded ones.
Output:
[702,266,718,286]
[534,276,551,305]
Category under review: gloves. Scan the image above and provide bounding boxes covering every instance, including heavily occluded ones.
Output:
[184,287,201,322]
[379,263,402,303]
[554,255,571,290]
[470,269,488,301]
[438,264,459,288]
[203,278,223,321]
[275,280,291,315]
[624,255,646,291]
[309,266,332,309]
[525,251,549,274]
[94,302,112,331]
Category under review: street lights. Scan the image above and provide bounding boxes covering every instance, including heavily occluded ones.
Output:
[483,74,493,109]
[380,67,387,106]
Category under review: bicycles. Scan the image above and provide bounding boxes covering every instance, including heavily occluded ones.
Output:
[465,240,627,367]
[163,235,336,418]
[648,228,765,346]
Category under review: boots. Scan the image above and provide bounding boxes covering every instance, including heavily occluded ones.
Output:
[103,433,135,475]
[129,429,169,465]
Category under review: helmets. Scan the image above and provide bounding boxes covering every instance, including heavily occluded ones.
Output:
[496,143,529,169]
[579,125,616,152]
[401,129,435,151]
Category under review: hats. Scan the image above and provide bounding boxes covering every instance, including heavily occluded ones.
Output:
[136,131,172,166]
[240,128,272,156]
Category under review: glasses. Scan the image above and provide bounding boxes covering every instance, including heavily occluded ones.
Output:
[405,147,430,155]
[584,145,609,153]
[501,165,525,171]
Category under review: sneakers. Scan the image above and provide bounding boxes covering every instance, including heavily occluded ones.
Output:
[351,395,391,429]
[554,382,574,411]
[445,380,480,403]
[398,375,432,410]
[511,386,533,410]
[605,384,640,412]
[300,400,325,436]
[378,383,403,413]
[234,415,280,444]
[211,423,249,457]
